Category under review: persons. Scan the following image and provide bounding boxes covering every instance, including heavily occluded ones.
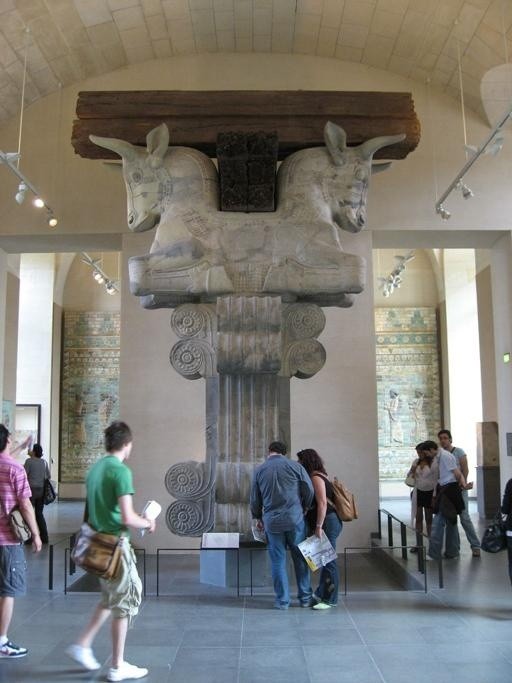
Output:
[422,440,466,559]
[63,420,156,682]
[436,429,483,558]
[498,478,512,586]
[408,387,428,446]
[249,441,320,611]
[24,442,52,545]
[406,442,434,552]
[383,388,404,448]
[297,447,343,610]
[0,422,42,658]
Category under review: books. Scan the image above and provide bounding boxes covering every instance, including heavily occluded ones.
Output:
[136,499,162,537]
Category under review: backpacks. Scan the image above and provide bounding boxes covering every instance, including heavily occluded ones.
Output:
[322,473,358,522]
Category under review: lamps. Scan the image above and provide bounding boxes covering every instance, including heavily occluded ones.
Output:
[434,106,510,222]
[0,147,59,228]
[79,250,121,296]
[374,247,416,299]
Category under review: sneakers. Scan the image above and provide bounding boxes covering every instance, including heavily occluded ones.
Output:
[66,644,101,670]
[301,597,312,607]
[473,549,480,557]
[0,641,28,658]
[410,547,419,552]
[106,661,148,682]
[426,554,435,561]
[313,602,332,610]
[279,600,290,610]
[312,593,322,603]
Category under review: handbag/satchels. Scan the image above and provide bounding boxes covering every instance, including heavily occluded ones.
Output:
[44,479,55,505]
[9,507,31,542]
[70,521,122,579]
[406,472,416,486]
[481,511,507,553]
